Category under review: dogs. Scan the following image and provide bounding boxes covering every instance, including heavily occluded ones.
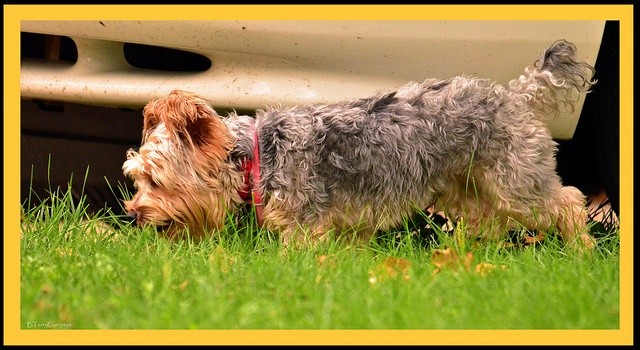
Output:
[122,38,598,258]
